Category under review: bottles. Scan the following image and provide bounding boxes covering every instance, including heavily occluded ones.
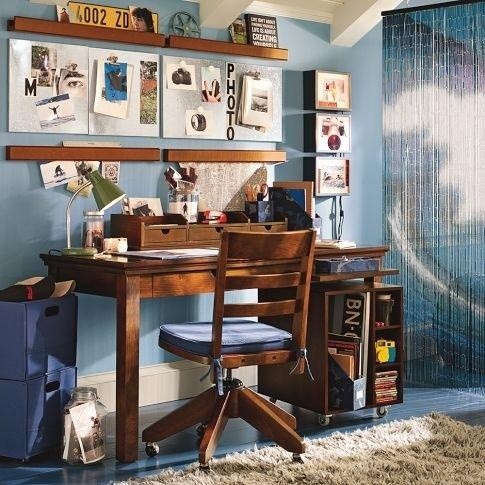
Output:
[58,386,108,468]
[81,210,104,253]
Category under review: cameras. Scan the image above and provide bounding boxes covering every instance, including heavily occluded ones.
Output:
[172,68,191,84]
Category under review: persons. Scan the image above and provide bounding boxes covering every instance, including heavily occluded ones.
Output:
[46,69,59,87]
[49,105,60,120]
[90,416,104,438]
[54,164,64,176]
[201,80,224,103]
[183,203,188,215]
[60,68,87,95]
[131,7,155,33]
[60,7,70,23]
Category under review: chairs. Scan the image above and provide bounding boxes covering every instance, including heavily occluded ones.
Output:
[142,227,317,470]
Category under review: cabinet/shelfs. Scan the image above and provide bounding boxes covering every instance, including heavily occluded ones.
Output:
[303,69,352,196]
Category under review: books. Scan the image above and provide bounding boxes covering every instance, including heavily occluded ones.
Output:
[374,368,399,405]
[315,239,357,249]
[327,331,363,381]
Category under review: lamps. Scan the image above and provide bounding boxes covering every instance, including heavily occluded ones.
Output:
[61,170,127,256]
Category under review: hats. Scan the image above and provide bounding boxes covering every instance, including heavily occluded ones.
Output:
[0,276,75,302]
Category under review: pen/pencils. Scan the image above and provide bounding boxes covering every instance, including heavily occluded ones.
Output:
[243,182,254,202]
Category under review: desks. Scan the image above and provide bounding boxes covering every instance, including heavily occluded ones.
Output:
[39,210,404,463]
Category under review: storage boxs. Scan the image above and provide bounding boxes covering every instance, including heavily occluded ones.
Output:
[0,366,78,463]
[0,291,78,382]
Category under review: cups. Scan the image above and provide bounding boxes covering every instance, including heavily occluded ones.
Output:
[168,194,198,224]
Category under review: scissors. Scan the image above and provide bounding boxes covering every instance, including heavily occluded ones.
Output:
[253,183,269,201]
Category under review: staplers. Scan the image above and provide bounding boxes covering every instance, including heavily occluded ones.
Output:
[202,211,227,224]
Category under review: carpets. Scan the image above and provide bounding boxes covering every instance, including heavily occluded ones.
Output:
[109,411,485,485]
